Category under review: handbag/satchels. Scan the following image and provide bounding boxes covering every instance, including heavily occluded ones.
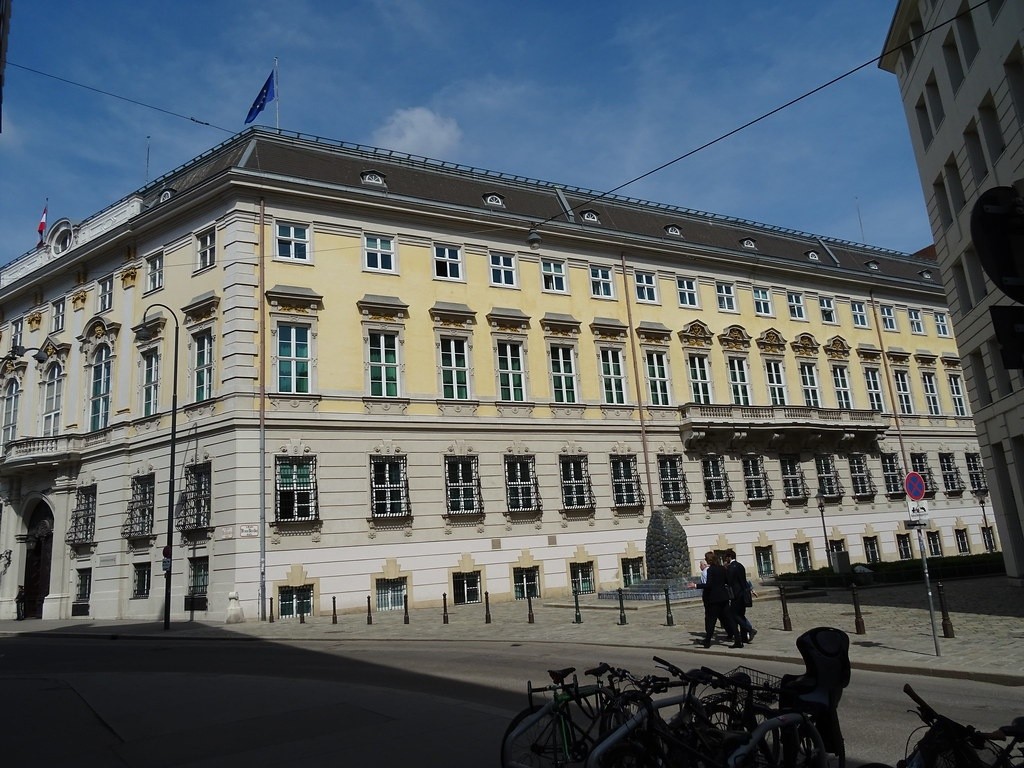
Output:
[725,584,734,599]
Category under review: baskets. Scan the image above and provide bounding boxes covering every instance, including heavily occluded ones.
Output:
[907,740,981,768]
[734,752,770,768]
[724,665,783,706]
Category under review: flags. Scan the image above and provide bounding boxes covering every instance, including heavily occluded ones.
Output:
[37,206,47,246]
[244,69,278,125]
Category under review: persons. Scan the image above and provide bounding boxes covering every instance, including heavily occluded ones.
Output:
[94,326,106,339]
[14,585,25,621]
[686,548,759,648]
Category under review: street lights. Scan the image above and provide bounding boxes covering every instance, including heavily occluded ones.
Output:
[136,301,180,629]
[974,481,993,555]
[814,487,835,572]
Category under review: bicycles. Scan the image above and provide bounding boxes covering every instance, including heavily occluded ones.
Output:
[498,656,846,768]
[853,682,1024,768]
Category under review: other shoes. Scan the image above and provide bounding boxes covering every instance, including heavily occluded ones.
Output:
[747,629,757,643]
[703,633,713,638]
[738,641,744,648]
[720,636,734,645]
[694,639,711,647]
